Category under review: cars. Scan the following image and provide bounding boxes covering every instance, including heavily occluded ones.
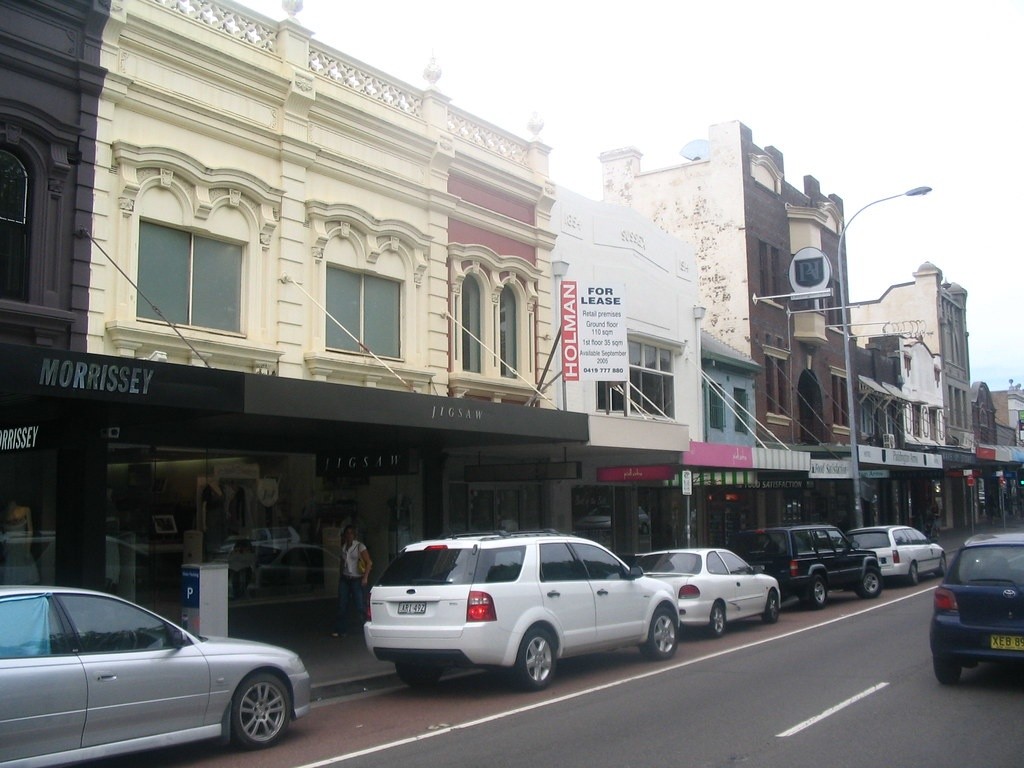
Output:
[838,523,947,587]
[927,532,1024,686]
[0,583,311,768]
[577,503,652,536]
[242,542,344,593]
[631,547,782,639]
[0,529,179,611]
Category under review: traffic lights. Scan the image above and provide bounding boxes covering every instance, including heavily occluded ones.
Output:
[1016,468,1024,486]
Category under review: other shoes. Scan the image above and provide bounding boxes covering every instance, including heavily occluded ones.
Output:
[331,633,341,637]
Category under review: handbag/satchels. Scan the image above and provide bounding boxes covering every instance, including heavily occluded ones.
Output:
[357,543,373,574]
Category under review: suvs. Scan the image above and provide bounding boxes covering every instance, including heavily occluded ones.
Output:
[361,526,682,692]
[727,523,885,611]
[220,525,306,559]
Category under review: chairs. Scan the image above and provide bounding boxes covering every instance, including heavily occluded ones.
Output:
[982,557,1009,569]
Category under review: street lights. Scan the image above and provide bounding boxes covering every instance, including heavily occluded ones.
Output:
[835,185,937,527]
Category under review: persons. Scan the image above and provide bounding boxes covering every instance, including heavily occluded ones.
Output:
[327,524,373,638]
[202,474,226,562]
[228,540,253,592]
[0,493,40,586]
[931,504,941,537]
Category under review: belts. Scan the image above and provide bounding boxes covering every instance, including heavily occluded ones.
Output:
[343,575,362,579]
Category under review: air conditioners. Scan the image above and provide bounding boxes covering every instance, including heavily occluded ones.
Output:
[883,434,895,448]
